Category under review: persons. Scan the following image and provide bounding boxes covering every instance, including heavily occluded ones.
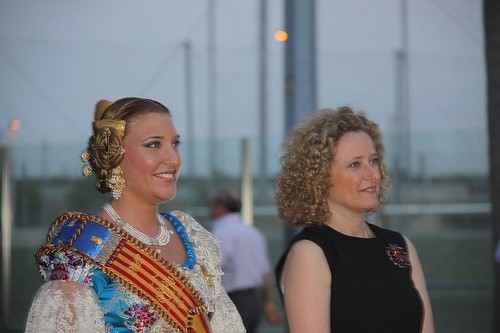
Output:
[25,97,247,332]
[274,105,436,332]
[207,185,280,332]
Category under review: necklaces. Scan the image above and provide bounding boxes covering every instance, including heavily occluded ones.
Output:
[101,201,174,253]
